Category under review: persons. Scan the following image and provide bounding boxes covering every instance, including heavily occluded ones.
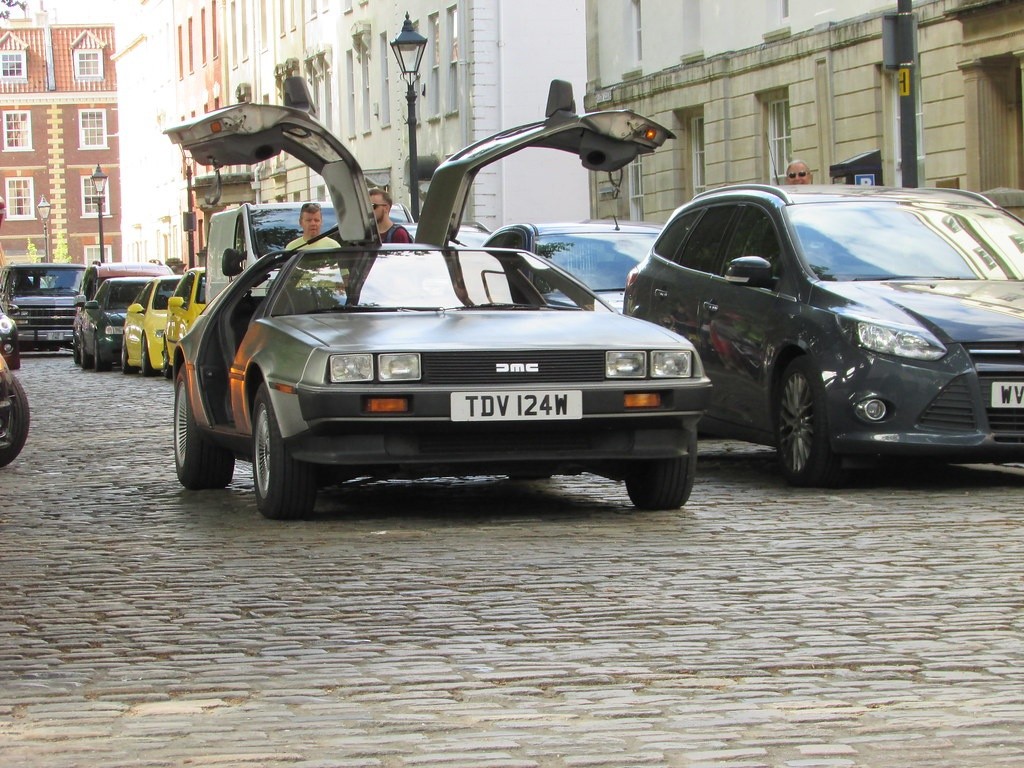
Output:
[785,161,813,185]
[285,203,341,250]
[368,189,413,243]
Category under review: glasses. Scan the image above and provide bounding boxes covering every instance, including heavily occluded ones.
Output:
[301,203,322,215]
[788,172,810,178]
[371,203,389,210]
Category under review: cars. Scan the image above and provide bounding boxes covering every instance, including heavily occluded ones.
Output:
[119,274,208,378]
[78,277,174,372]
[0,294,22,371]
[163,266,236,379]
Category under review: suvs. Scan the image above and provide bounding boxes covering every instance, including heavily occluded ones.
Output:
[398,222,495,248]
[478,217,691,315]
[0,261,88,350]
[72,259,177,365]
[621,183,1024,488]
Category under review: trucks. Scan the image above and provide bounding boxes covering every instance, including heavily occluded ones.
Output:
[204,201,417,307]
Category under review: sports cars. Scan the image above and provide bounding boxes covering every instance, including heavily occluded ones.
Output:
[159,77,714,524]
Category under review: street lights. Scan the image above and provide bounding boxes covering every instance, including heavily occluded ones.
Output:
[182,150,198,270]
[36,194,53,284]
[90,161,109,264]
[389,9,429,223]
